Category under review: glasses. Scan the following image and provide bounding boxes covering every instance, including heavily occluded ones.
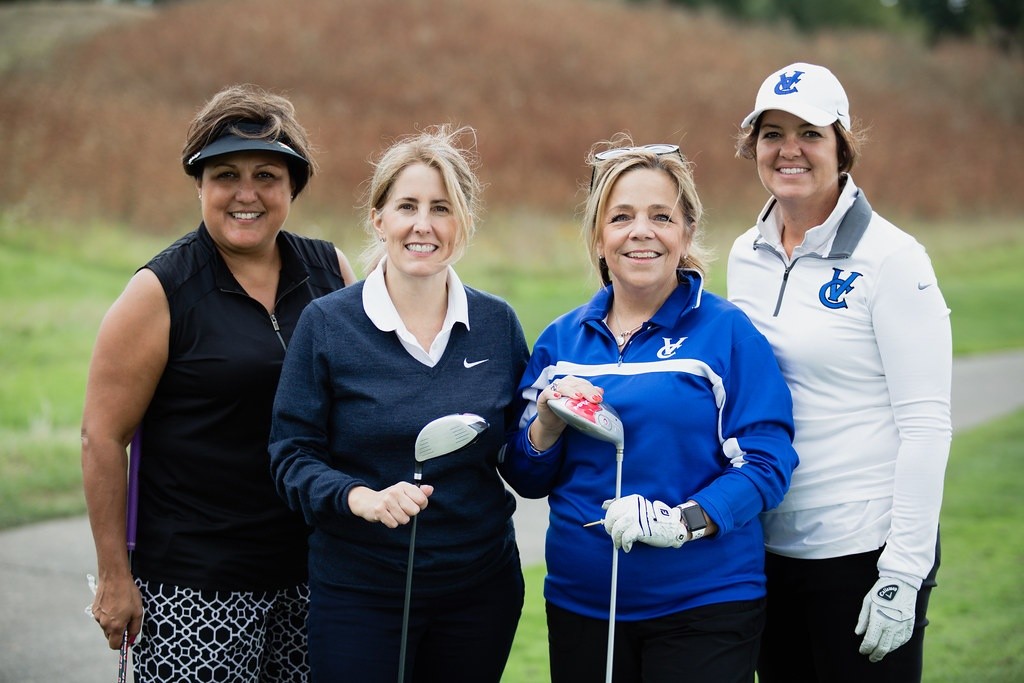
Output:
[590,142,685,204]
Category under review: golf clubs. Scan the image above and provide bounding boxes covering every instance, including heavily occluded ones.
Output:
[397,413,488,683]
[549,394,626,683]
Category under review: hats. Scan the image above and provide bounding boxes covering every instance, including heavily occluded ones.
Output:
[184,120,310,172]
[741,63,852,134]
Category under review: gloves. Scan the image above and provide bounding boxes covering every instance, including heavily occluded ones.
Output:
[602,493,688,554]
[855,576,917,661]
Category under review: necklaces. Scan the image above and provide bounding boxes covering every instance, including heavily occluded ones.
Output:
[613,309,647,347]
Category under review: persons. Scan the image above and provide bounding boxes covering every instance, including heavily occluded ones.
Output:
[725,62,951,682]
[81,82,363,683]
[502,146,802,683]
[262,135,530,683]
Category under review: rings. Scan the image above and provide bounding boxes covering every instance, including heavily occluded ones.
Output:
[551,382,558,391]
